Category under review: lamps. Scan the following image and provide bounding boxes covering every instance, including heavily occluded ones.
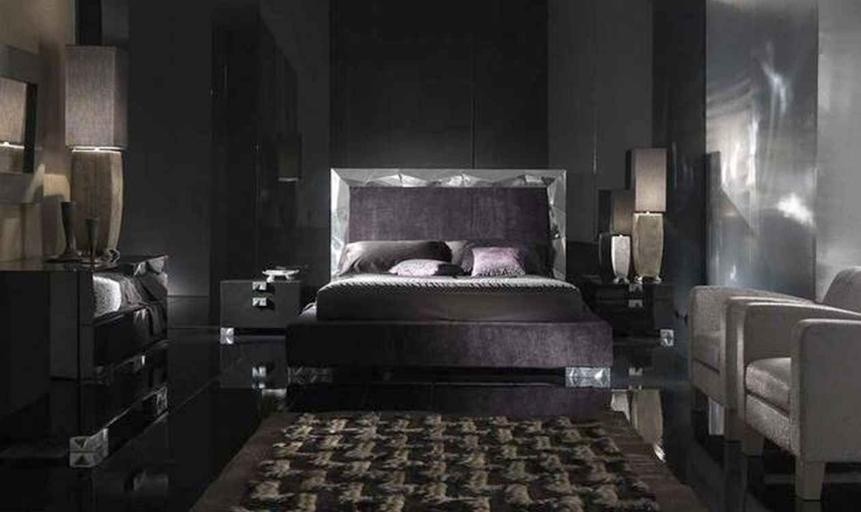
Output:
[608,188,633,285]
[61,42,130,253]
[625,146,668,280]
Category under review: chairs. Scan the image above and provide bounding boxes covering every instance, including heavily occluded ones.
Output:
[735,301,861,502]
[686,266,860,443]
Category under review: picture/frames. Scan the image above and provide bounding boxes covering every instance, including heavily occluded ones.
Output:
[1,41,49,205]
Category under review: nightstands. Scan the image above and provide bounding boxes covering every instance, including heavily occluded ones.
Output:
[577,273,672,338]
[218,278,305,347]
[218,335,289,391]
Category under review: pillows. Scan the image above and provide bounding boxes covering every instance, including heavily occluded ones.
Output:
[332,239,452,277]
[385,259,463,277]
[469,246,529,278]
[443,239,473,274]
[462,239,526,272]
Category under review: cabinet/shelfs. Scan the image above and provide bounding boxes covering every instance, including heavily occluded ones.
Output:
[0,252,172,470]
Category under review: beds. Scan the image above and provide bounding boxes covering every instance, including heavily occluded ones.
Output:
[283,167,614,390]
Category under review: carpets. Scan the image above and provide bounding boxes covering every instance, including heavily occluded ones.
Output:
[187,408,710,511]
